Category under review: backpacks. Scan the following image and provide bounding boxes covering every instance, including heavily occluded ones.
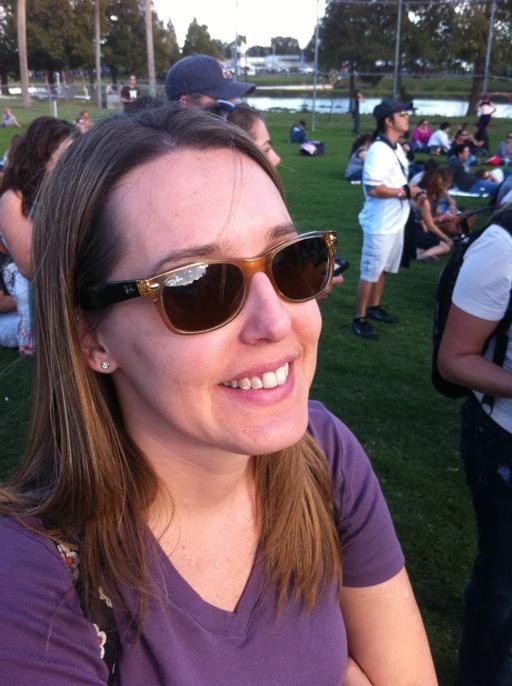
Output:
[431,202,512,398]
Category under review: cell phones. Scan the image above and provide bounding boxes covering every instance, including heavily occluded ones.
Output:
[333,256,349,276]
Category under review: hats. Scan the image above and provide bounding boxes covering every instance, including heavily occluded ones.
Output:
[166,56,254,101]
[373,98,412,120]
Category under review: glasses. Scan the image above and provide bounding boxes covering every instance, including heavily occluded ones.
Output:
[79,230,337,335]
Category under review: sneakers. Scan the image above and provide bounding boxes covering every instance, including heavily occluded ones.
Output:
[367,306,399,323]
[352,317,378,339]
